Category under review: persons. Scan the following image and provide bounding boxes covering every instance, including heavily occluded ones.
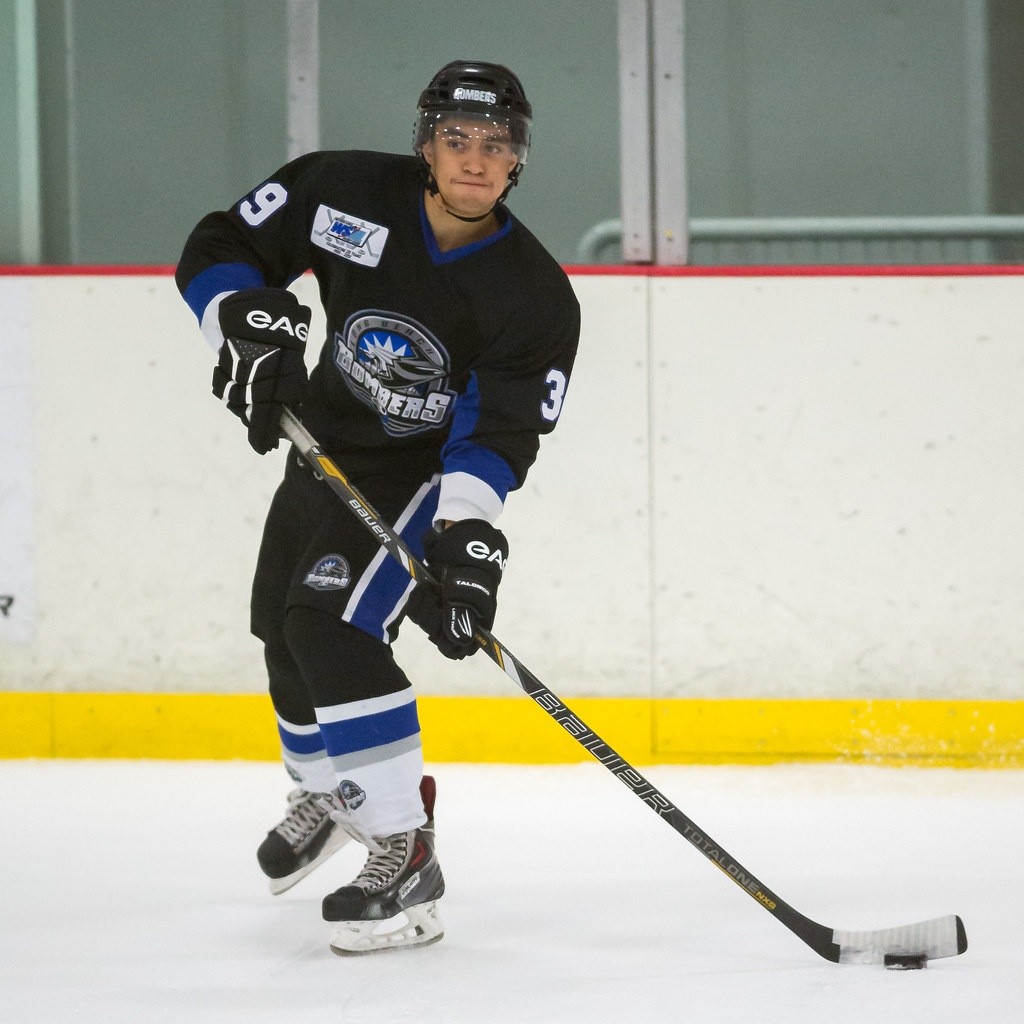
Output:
[174,58,581,951]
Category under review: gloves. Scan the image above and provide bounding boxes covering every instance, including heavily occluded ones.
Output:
[212,287,311,453]
[407,518,509,660]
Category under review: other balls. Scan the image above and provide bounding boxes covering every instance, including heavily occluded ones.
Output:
[882,953,930,971]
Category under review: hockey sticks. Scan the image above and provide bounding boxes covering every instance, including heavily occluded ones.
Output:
[271,404,976,965]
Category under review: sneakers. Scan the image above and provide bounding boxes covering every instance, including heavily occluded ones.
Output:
[321,775,445,952]
[257,789,352,896]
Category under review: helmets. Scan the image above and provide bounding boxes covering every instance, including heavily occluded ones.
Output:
[419,59,534,143]
